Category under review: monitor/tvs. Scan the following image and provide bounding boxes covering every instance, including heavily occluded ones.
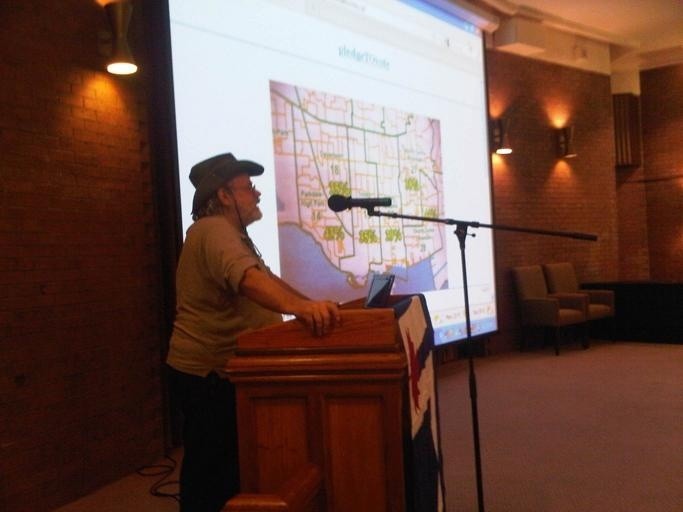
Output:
[364,272,396,307]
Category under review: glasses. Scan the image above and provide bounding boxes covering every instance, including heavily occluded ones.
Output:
[231,183,256,195]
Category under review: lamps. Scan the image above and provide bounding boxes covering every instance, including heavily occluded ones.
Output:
[99,1,139,77]
[492,118,578,160]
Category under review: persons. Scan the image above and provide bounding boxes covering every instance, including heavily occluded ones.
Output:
[163,153,340,511]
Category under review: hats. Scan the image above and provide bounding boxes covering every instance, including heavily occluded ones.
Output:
[186,153,265,213]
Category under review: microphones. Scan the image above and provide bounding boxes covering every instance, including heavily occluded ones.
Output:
[327,194,392,211]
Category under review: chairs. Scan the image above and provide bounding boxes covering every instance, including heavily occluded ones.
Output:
[510,260,615,355]
